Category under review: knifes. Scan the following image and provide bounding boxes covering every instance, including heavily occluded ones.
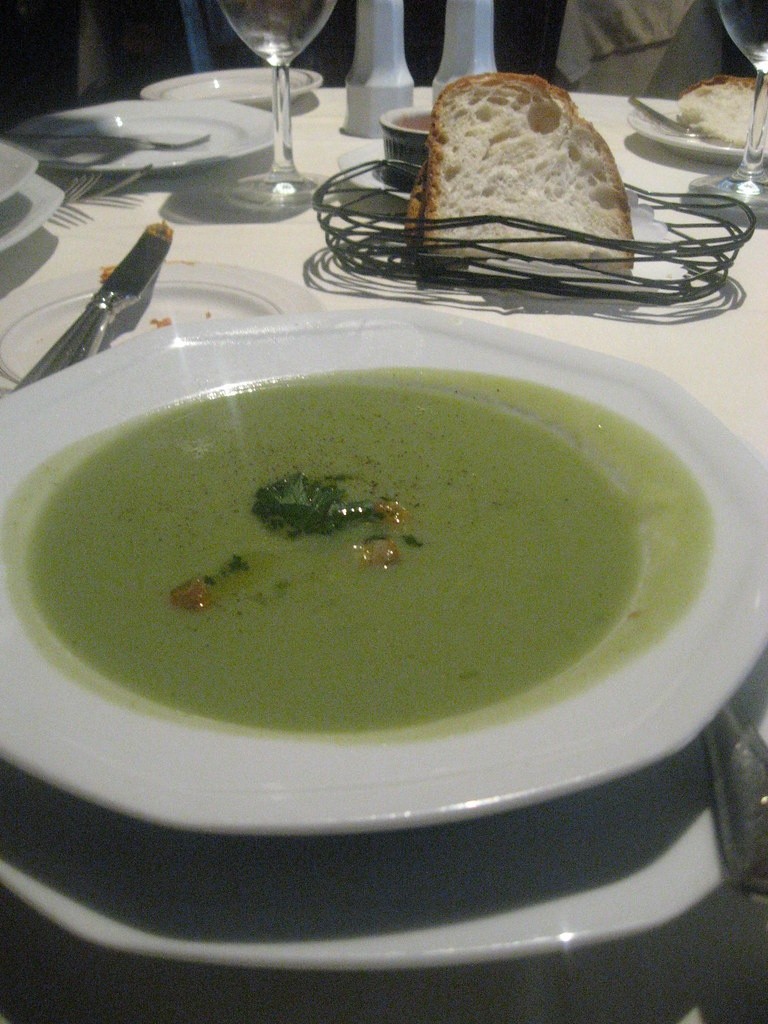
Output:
[8,224,174,412]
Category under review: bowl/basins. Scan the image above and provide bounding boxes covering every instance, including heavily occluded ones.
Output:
[379,106,435,174]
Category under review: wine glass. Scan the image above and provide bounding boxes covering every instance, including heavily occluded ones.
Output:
[688,0,768,221]
[216,1,340,208]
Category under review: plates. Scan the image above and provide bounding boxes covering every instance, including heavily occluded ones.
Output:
[0,141,39,203]
[0,654,767,969]
[0,175,66,261]
[0,303,767,835]
[0,261,328,399]
[629,97,768,168]
[2,100,278,173]
[139,65,324,115]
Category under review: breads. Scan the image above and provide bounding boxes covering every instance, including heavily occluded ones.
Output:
[406,72,634,285]
[677,75,767,147]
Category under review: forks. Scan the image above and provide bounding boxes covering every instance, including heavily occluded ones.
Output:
[82,163,151,200]
[63,171,103,203]
[2,129,211,152]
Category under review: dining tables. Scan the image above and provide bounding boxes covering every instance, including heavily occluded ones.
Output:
[0,89,768,1024]
